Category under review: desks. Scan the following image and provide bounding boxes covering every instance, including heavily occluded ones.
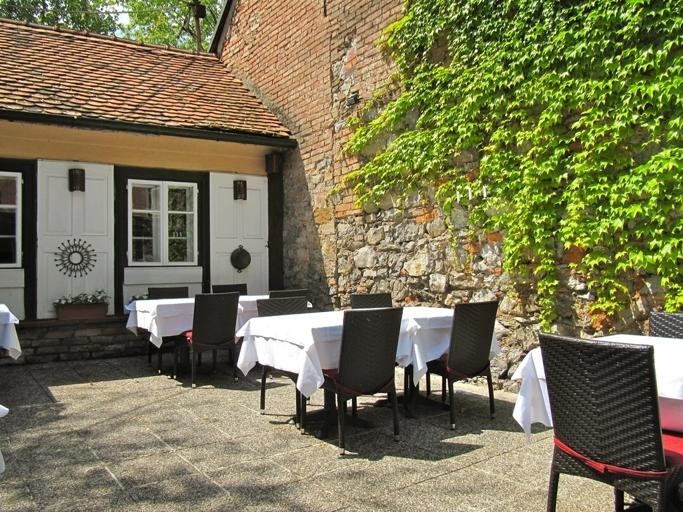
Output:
[235,305,499,438]
[532,333,682,435]
[133,292,308,373]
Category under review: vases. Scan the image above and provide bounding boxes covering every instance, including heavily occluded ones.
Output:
[55,304,108,319]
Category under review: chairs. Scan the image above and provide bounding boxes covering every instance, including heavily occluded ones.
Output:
[350,293,393,309]
[149,286,190,371]
[299,308,403,454]
[404,300,499,429]
[211,284,249,297]
[539,313,682,512]
[258,296,306,416]
[181,293,240,371]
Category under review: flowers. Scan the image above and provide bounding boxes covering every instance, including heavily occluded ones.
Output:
[55,288,112,305]
